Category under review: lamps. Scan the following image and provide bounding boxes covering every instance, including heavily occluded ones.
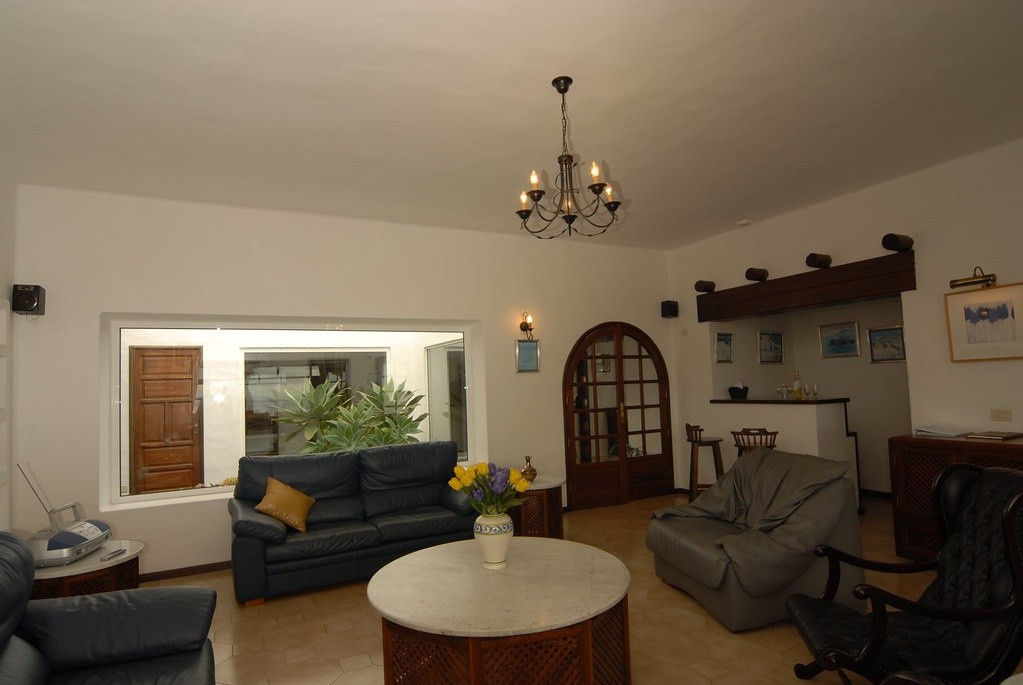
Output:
[520,312,534,342]
[514,75,621,240]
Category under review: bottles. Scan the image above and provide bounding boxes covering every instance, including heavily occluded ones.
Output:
[521,456,537,482]
[792,370,801,399]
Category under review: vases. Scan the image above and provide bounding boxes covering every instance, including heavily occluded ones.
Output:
[521,456,537,482]
[474,512,514,570]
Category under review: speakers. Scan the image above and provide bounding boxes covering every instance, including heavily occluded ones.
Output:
[661,300,679,318]
[12,285,46,315]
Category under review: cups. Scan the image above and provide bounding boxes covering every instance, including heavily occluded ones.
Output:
[776,383,792,400]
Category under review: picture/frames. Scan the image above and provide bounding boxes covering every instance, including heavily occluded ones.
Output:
[757,330,784,364]
[944,281,1023,364]
[715,332,734,363]
[818,320,860,359]
[867,325,907,365]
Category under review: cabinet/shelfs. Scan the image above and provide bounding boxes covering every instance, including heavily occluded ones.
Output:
[888,434,1023,581]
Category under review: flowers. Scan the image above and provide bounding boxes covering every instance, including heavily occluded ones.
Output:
[448,462,532,515]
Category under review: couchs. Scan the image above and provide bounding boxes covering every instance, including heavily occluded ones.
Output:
[0,531,217,685]
[646,446,867,634]
[228,440,481,607]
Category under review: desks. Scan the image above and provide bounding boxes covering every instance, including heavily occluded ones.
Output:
[506,474,566,540]
[31,539,144,599]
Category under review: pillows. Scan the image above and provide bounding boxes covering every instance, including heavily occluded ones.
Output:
[254,477,316,533]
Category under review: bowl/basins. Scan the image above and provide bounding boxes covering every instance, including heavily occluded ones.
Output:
[728,386,749,399]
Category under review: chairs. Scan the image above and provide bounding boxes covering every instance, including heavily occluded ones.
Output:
[784,463,1023,685]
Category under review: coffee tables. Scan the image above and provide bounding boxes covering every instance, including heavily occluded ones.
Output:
[367,537,631,685]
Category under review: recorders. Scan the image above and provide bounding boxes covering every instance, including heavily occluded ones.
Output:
[14,462,113,569]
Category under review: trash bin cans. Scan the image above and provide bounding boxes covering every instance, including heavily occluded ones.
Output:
[606,410,629,456]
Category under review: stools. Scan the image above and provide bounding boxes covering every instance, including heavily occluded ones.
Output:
[686,423,724,503]
[731,428,778,458]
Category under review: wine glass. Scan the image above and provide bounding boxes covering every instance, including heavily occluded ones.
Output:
[803,383,810,398]
[813,383,819,398]
